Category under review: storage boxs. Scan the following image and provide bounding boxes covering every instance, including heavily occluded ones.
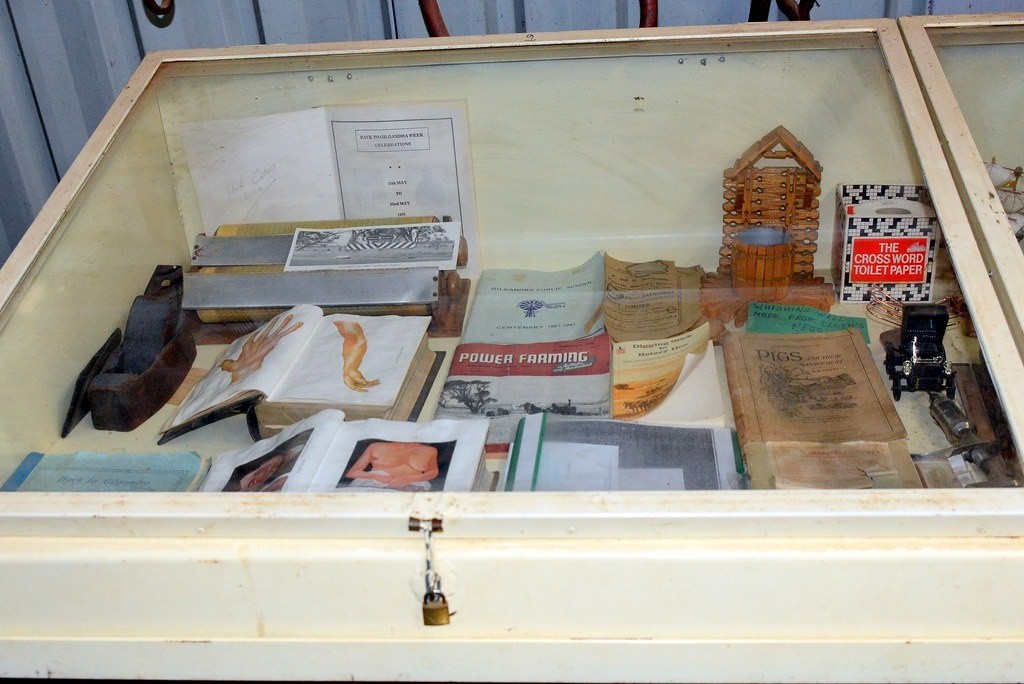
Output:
[829,184,942,304]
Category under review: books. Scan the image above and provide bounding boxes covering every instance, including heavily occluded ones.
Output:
[0,251,924,493]
[182,97,484,281]
